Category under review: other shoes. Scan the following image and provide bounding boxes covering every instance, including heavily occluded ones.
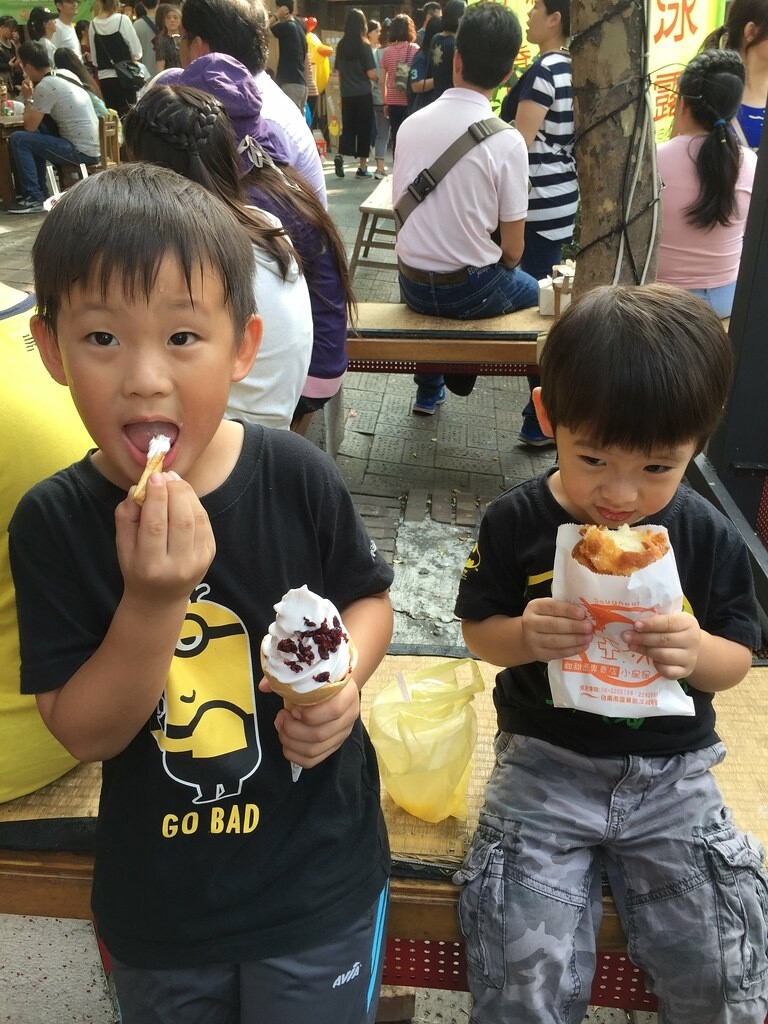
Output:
[355,166,372,179]
[335,154,345,178]
[374,170,386,181]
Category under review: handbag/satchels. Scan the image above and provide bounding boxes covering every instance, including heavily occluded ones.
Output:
[395,43,412,94]
[113,60,151,92]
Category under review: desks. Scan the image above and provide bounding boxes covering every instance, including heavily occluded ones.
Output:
[0,121,26,210]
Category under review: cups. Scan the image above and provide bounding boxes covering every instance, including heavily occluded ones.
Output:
[372,679,466,770]
[43,192,66,212]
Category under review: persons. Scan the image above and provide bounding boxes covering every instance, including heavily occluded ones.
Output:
[155,53,358,418]
[1,279,97,805]
[268,0,319,120]
[393,2,552,446]
[179,0,327,210]
[455,284,768,1024]
[703,0,768,154]
[333,1,466,180]
[656,51,757,332]
[0,0,181,213]
[123,85,313,431]
[501,0,579,279]
[7,162,395,1024]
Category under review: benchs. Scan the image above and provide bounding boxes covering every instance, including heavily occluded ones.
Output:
[1,646,768,1014]
[346,174,400,283]
[303,303,559,461]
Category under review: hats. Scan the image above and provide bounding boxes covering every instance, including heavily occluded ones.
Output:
[30,7,60,23]
[146,52,294,182]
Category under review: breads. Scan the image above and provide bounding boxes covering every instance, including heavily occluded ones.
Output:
[571,522,668,578]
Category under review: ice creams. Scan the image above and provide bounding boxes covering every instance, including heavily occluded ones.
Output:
[261,584,358,711]
[131,434,172,505]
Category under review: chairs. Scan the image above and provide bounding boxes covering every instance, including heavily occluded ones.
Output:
[59,113,121,195]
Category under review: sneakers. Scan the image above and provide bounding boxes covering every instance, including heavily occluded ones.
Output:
[6,194,45,213]
[412,385,446,413]
[516,414,555,447]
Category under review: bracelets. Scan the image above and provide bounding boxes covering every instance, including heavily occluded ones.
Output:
[274,14,279,21]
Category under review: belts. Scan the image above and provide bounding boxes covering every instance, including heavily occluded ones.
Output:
[398,256,470,286]
